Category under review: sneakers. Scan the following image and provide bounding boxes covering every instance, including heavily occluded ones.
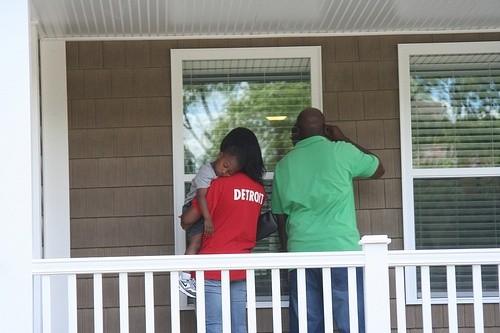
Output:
[179,277,197,298]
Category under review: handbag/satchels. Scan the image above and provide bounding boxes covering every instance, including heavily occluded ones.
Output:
[256,192,278,241]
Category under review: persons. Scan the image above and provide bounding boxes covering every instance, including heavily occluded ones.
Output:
[181,127,267,333]
[271,108,385,333]
[177,144,248,299]
